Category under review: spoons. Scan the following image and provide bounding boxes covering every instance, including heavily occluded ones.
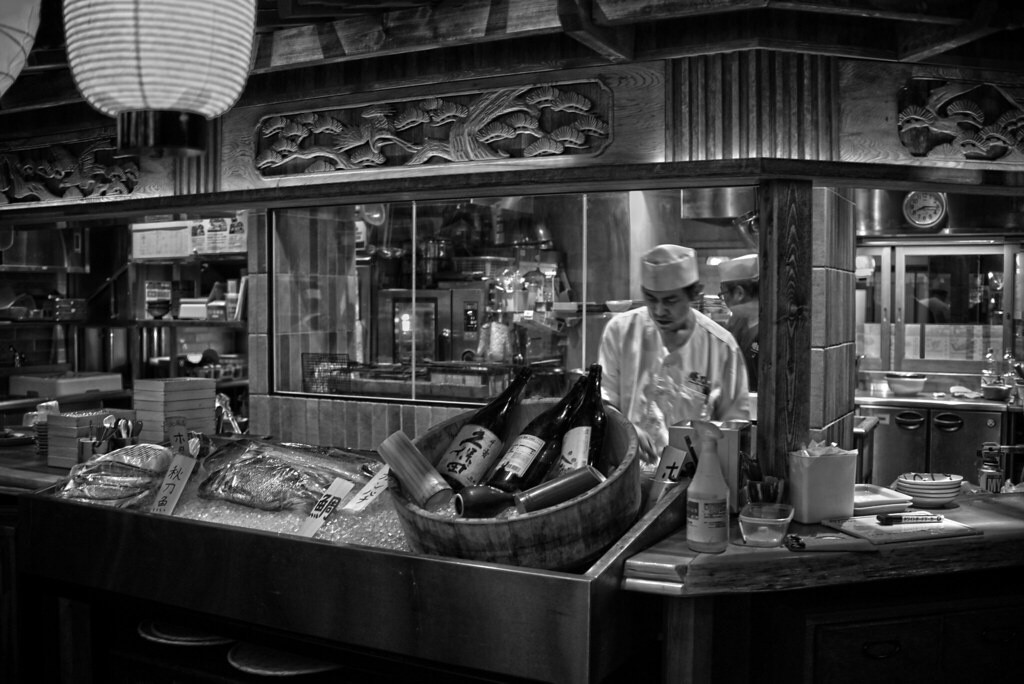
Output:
[115,418,143,438]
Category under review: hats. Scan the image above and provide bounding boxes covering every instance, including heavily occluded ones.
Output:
[639,244,699,292]
[716,254,759,282]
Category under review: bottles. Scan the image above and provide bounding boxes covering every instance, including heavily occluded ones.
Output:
[981,348,1016,396]
[451,487,519,519]
[488,376,590,495]
[433,367,535,492]
[558,363,605,476]
[204,281,237,321]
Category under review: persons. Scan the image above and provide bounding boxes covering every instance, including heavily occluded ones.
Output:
[718,254,760,392]
[918,277,949,324]
[473,281,528,397]
[596,244,751,486]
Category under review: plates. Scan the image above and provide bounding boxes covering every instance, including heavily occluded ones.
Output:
[896,472,964,507]
[848,483,915,514]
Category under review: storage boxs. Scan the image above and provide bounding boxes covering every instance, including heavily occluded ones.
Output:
[128,220,196,259]
[789,446,858,523]
[737,501,794,543]
[452,255,515,278]
[9,372,216,469]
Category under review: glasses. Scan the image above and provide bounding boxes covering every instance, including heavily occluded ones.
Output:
[717,289,733,301]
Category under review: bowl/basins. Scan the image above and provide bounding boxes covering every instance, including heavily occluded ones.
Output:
[606,299,632,312]
[739,500,795,547]
[884,371,927,396]
[387,397,643,571]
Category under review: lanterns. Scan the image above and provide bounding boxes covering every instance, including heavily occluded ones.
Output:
[64,0,256,159]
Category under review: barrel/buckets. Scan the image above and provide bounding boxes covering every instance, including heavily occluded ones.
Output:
[790,447,857,524]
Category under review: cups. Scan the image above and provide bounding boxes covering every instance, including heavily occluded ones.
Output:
[375,428,454,511]
[77,437,107,459]
[113,434,139,446]
[512,467,606,515]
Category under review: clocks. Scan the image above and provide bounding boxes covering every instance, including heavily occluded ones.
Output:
[902,189,948,229]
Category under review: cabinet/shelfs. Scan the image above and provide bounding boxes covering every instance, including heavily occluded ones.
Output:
[859,405,1003,486]
